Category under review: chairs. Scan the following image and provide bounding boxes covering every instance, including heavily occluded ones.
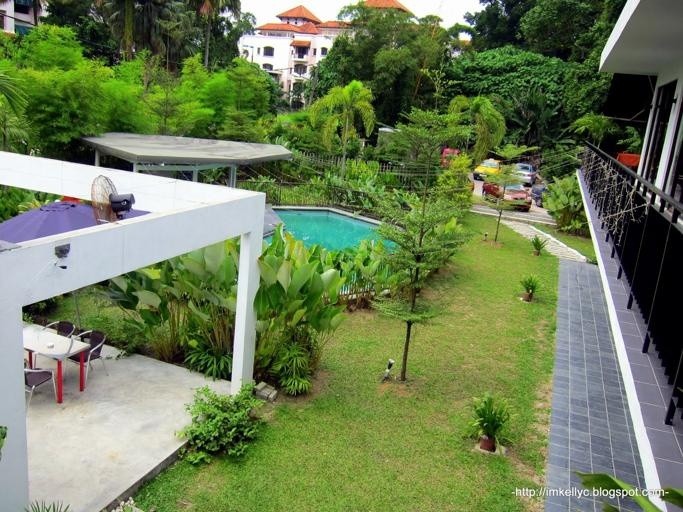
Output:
[23,321,108,409]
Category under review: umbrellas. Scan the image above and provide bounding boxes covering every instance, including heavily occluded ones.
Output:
[0,201,151,244]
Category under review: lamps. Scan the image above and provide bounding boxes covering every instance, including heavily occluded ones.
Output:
[55,245,71,271]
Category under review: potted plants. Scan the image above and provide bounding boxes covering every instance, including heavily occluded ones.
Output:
[472,398,510,458]
[519,235,549,302]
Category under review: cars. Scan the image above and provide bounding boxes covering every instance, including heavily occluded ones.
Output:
[439,147,550,214]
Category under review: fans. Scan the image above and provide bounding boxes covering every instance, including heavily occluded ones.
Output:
[91,175,135,226]
